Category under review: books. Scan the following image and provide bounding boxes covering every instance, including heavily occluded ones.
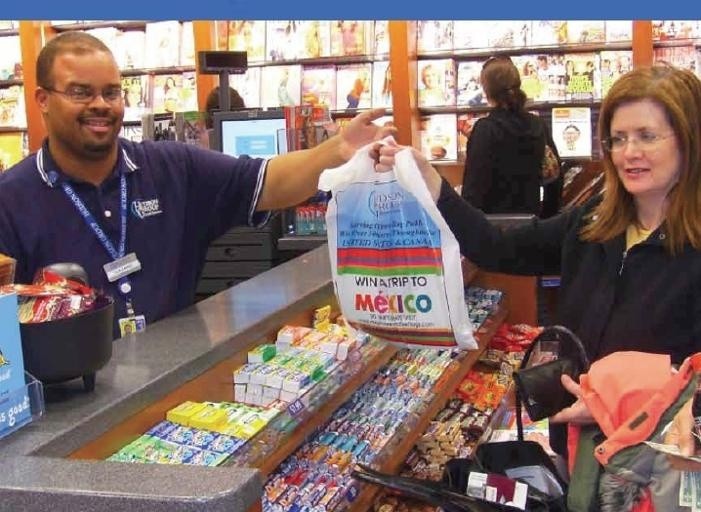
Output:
[652,20,700,76]
[1,20,30,165]
[93,20,198,143]
[417,21,632,50]
[217,20,393,132]
[416,60,488,107]
[515,52,632,100]
[551,107,592,156]
[419,113,490,163]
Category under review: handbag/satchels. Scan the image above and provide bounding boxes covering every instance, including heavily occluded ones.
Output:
[537,143,561,185]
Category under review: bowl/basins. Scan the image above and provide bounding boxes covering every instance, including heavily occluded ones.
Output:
[19,294,115,391]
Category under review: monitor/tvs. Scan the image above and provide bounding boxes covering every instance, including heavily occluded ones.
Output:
[216,111,288,160]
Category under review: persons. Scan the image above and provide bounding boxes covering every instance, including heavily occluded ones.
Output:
[376,66,701,484]
[0,31,397,340]
[461,57,563,340]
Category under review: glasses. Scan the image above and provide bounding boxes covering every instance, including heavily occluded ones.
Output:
[42,84,126,103]
[602,130,680,153]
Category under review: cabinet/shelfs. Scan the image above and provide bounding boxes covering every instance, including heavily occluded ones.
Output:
[66,254,540,512]
[0,19,701,201]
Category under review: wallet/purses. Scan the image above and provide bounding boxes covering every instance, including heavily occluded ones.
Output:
[512,354,580,422]
